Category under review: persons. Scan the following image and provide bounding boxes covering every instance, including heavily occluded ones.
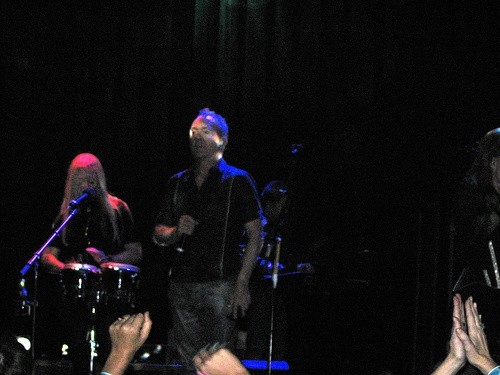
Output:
[152,108,266,375]
[239,180,315,360]
[39,153,145,359]
[99,311,250,375]
[451,127,500,375]
[428,292,500,375]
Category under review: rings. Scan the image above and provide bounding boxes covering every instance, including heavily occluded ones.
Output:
[479,322,485,329]
[117,317,123,322]
[462,322,466,327]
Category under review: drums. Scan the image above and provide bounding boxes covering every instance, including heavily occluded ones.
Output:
[100,262,141,311]
[66,263,104,312]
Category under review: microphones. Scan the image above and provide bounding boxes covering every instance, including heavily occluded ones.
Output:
[69,188,95,209]
[176,208,198,255]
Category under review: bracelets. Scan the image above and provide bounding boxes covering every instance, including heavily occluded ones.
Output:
[99,371,113,375]
